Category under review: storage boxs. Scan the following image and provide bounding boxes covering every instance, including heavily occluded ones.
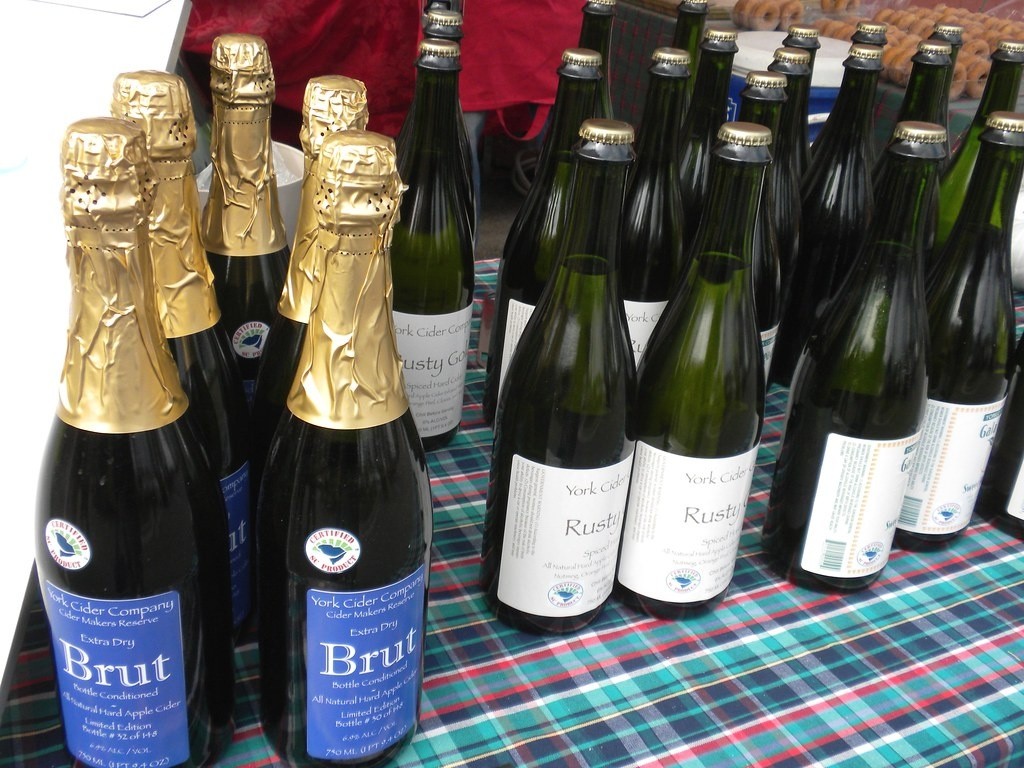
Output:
[724,29,858,145]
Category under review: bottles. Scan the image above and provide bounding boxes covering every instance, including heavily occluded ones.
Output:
[34,1,1024,768]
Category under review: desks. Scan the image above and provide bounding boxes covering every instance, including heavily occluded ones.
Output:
[0,256,1024,768]
